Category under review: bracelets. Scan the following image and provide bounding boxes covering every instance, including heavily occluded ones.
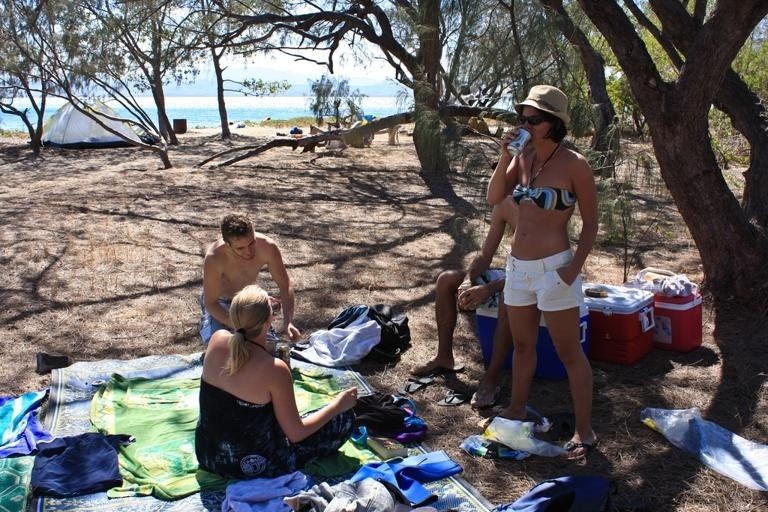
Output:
[488,281,496,298]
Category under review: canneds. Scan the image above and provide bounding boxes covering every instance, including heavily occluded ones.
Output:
[507,130,531,158]
[280,345,291,369]
[265,341,276,355]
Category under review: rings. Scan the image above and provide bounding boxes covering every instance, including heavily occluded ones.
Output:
[474,303,478,309]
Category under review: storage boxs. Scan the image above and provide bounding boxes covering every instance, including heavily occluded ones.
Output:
[622,290,703,353]
[476,302,590,381]
[581,282,655,365]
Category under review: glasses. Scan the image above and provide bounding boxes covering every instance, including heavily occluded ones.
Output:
[518,114,552,126]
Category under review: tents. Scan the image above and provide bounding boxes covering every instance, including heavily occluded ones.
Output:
[31,95,143,149]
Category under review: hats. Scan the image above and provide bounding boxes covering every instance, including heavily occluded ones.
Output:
[514,85,571,127]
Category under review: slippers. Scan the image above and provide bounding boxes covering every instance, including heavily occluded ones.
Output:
[471,384,500,408]
[409,358,465,374]
[560,436,599,460]
[397,376,434,396]
[483,411,528,432]
[437,389,470,406]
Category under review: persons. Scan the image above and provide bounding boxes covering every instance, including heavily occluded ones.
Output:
[407,163,522,410]
[486,83,602,467]
[201,211,301,363]
[194,283,360,481]
